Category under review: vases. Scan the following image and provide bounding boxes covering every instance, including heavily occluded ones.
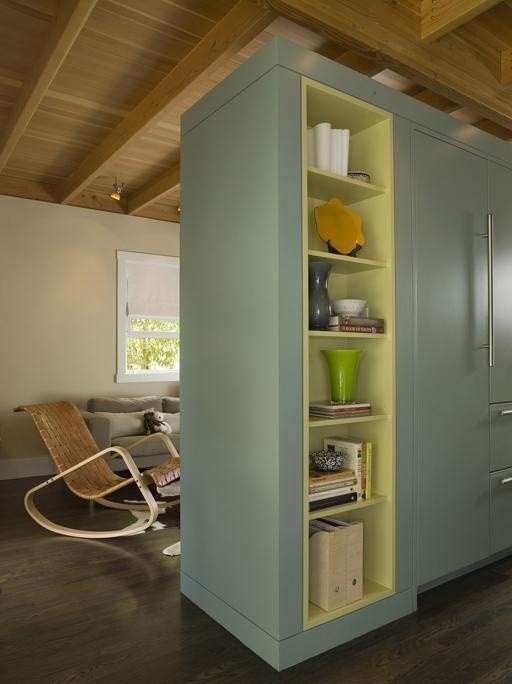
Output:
[320,347,368,406]
[309,261,336,330]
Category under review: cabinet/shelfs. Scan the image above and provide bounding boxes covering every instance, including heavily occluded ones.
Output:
[181,35,512,672]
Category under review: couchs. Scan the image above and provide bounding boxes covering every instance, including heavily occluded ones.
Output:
[80,395,180,513]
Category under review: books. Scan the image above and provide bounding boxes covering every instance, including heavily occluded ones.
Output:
[327,315,386,333]
[309,435,373,511]
[309,401,372,418]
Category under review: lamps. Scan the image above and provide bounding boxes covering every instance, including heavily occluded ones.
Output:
[109,177,129,202]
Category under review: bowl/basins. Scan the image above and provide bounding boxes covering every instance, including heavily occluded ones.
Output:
[331,298,369,318]
[311,450,346,474]
[348,171,370,184]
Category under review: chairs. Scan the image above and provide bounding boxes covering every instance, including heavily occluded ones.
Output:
[13,399,181,538]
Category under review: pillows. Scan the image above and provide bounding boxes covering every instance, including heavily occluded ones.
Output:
[93,407,181,440]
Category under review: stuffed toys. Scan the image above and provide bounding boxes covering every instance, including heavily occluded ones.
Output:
[144,411,174,434]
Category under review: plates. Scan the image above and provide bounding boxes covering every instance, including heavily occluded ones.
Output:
[312,195,367,256]
